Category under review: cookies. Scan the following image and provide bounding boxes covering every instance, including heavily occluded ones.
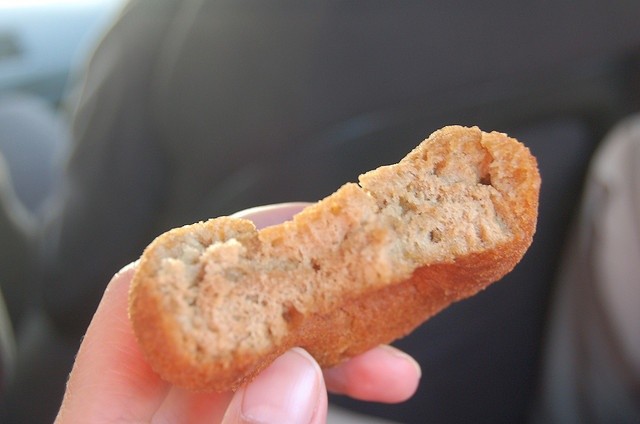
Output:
[128,125,543,395]
